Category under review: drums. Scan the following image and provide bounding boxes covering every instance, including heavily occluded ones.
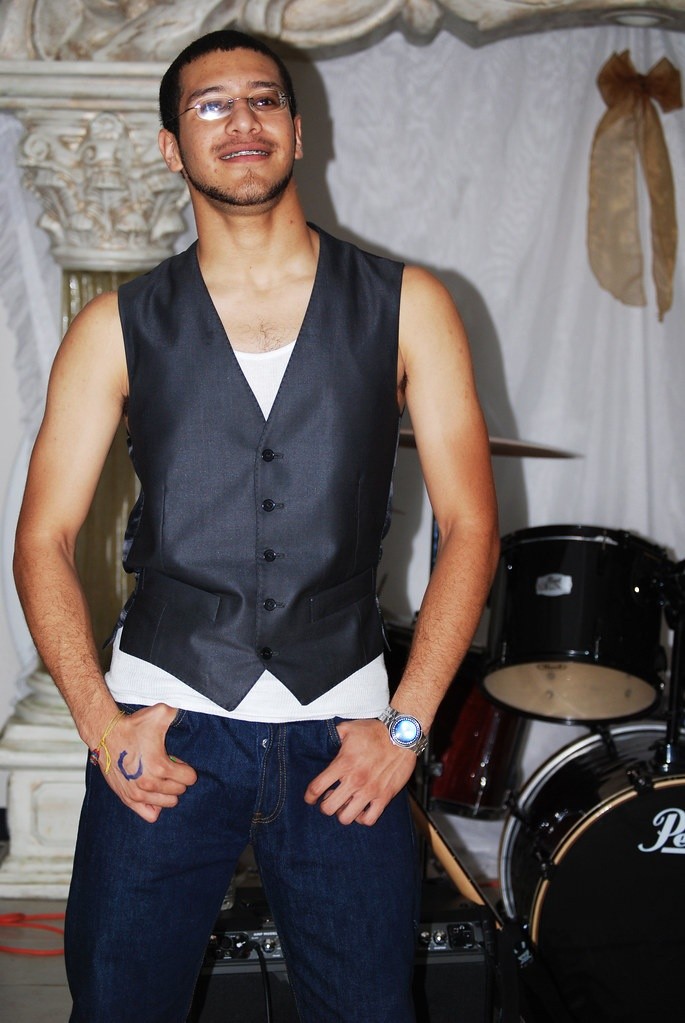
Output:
[478,522,677,725]
[496,722,685,1016]
[383,609,528,827]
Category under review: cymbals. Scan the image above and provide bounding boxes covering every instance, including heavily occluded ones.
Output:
[398,424,579,463]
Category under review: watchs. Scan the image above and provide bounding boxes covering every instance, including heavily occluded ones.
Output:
[377,704,429,755]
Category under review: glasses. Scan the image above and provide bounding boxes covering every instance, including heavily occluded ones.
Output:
[163,92,293,125]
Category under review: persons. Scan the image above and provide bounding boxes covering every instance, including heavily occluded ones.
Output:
[14,30,501,1023]
